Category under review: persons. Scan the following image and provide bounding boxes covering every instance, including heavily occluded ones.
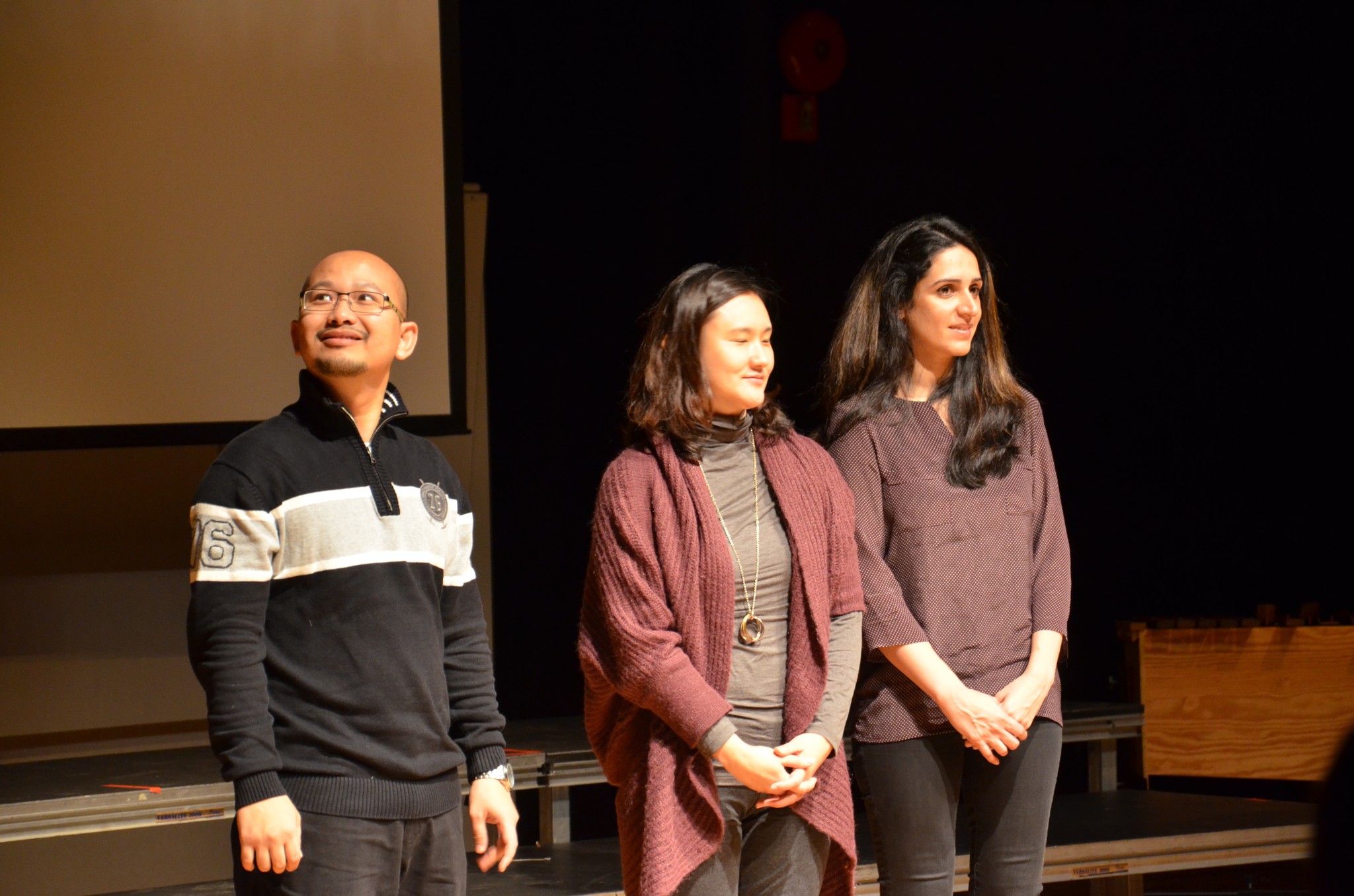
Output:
[184,250,523,896]
[576,261,865,896]
[816,215,1072,896]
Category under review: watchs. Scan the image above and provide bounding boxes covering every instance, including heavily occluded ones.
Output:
[470,763,515,792]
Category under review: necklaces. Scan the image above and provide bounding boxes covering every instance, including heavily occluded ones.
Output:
[694,427,766,646]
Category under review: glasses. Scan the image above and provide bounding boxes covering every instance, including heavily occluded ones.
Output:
[299,288,405,323]
